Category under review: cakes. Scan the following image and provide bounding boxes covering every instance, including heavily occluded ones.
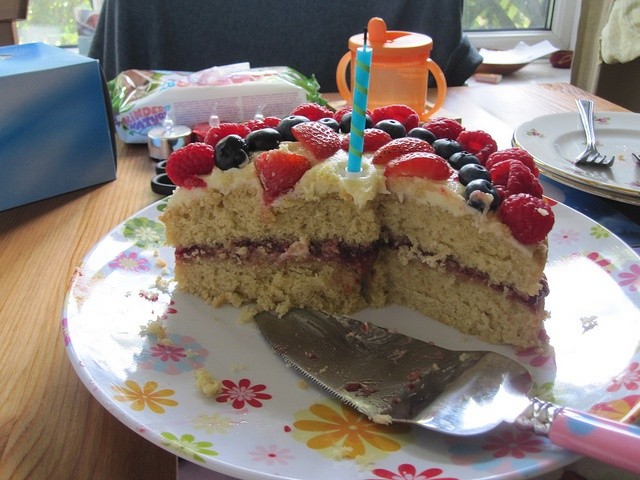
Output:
[160,102,555,352]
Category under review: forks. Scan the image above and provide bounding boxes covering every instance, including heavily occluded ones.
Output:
[574,99,616,167]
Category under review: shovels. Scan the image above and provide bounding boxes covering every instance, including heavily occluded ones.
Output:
[253,302,640,480]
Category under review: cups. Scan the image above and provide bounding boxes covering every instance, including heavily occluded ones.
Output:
[336,16,447,124]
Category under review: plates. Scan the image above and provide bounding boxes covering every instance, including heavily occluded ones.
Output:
[513,110,640,198]
[61,194,640,480]
[511,139,640,210]
